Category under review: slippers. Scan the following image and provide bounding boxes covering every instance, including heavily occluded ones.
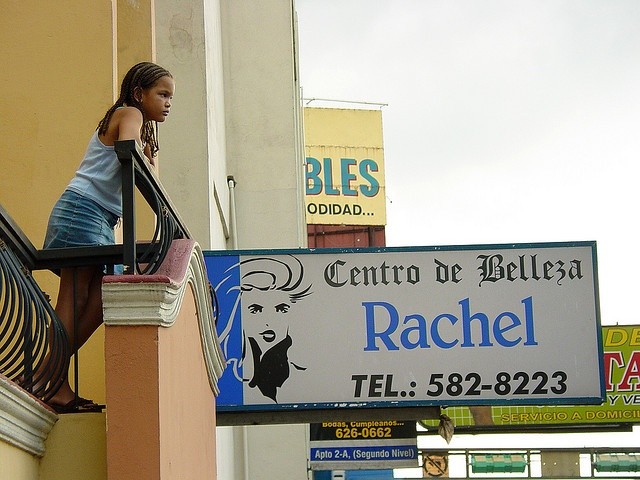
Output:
[45,396,103,416]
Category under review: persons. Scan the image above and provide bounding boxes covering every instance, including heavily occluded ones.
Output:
[22,59,173,411]
